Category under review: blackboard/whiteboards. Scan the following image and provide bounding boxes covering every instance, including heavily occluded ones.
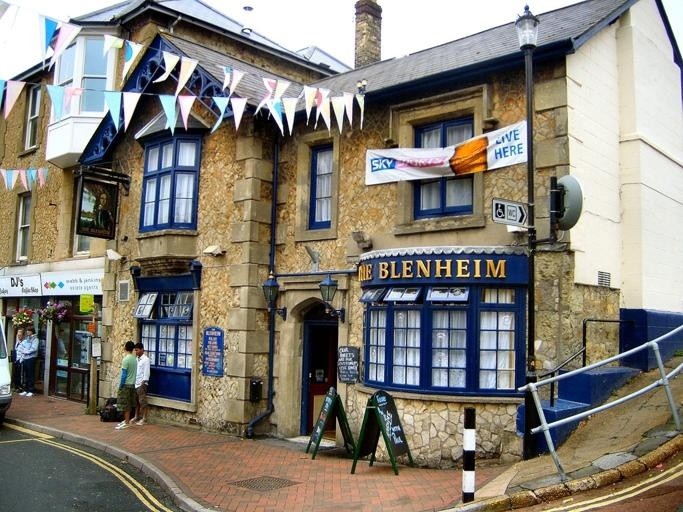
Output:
[372,390,409,457]
[356,390,380,458]
[336,395,355,446]
[310,394,335,445]
[338,345,360,384]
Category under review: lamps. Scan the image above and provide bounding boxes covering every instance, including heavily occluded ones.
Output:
[318,274,344,324]
[261,269,286,321]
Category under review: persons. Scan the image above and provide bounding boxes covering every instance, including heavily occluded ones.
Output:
[18,326,39,398]
[13,330,28,394]
[130,344,151,425]
[115,341,131,430]
[92,189,115,232]
[56,329,67,360]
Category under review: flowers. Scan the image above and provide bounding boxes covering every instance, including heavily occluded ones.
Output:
[11,297,67,329]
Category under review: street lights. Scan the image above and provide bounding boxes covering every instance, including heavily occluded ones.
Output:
[513,2,541,460]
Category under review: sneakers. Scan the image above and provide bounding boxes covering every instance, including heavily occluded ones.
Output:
[12,387,34,397]
[115,417,146,430]
